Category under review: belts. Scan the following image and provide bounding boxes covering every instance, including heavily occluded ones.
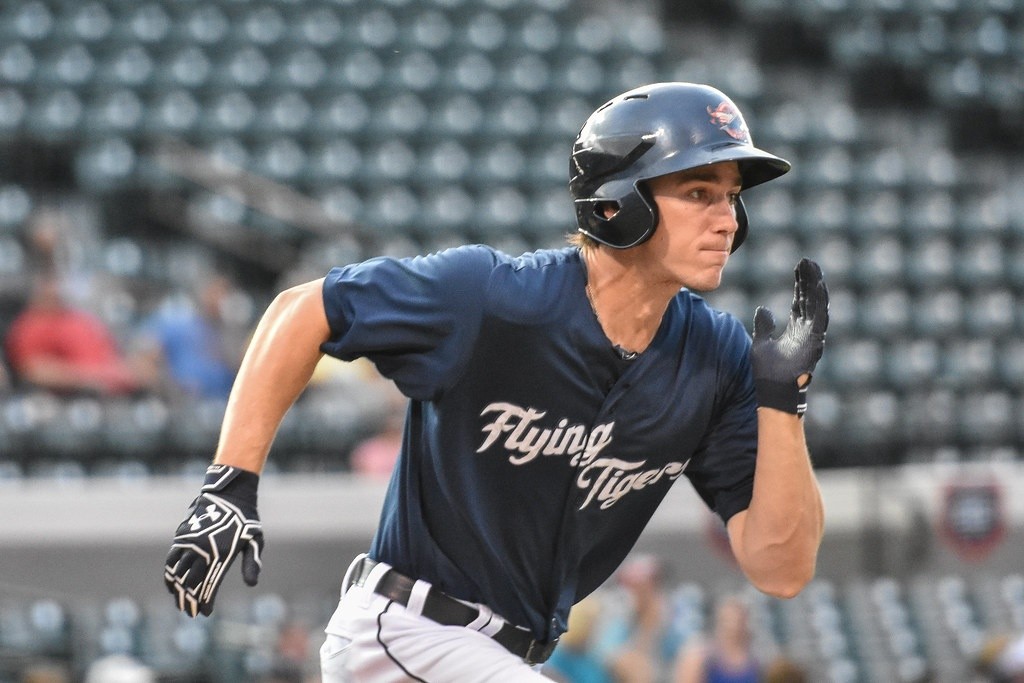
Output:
[350,554,560,667]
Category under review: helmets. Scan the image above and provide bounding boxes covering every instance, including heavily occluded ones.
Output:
[570,81,791,255]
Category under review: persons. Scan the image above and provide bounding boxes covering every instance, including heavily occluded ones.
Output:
[349,400,403,475]
[0,200,257,400]
[975,631,1024,683]
[8,647,162,683]
[165,82,829,683]
[542,554,809,683]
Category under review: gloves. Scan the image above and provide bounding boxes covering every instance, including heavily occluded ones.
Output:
[750,257,830,418]
[164,464,265,618]
